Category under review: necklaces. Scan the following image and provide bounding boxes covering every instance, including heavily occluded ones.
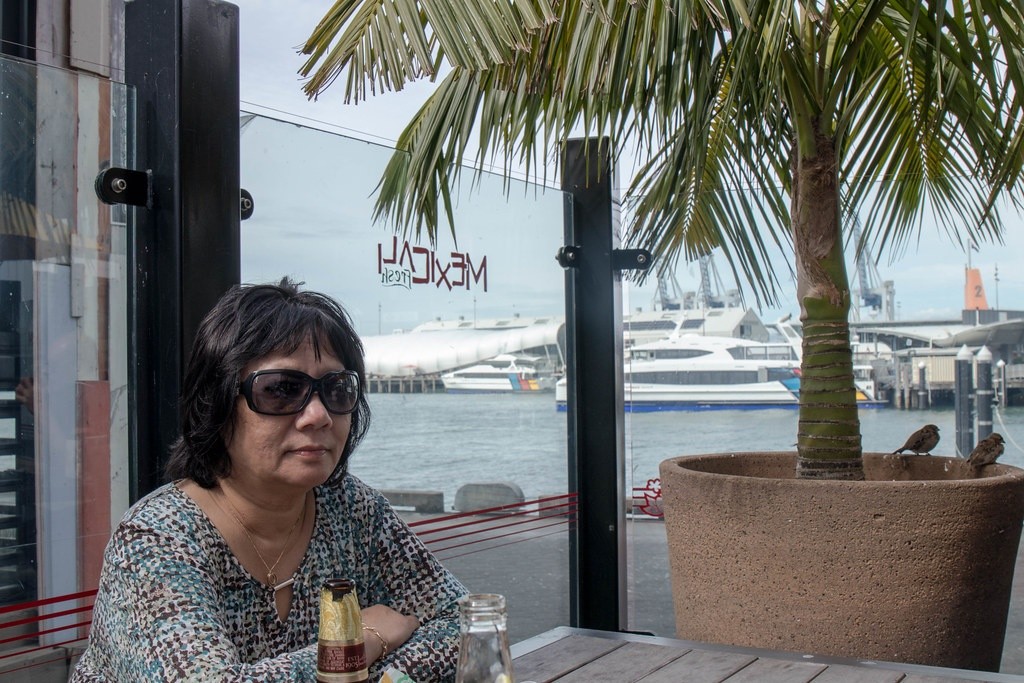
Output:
[219,492,304,584]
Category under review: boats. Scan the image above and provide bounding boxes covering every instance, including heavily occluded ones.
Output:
[351,316,894,415]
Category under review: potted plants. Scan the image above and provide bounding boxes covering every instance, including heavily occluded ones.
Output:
[298,1,1024,672]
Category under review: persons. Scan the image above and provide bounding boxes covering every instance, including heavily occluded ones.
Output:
[68,275,474,683]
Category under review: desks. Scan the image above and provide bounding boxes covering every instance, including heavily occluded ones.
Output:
[504,625,1024,683]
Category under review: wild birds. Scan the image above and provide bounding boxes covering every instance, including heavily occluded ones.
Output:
[891,424,940,455]
[960,433,1006,469]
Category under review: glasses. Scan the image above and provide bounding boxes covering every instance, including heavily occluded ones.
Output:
[238,368,361,415]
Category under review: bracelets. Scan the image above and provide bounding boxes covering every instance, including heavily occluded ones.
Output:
[361,623,388,663]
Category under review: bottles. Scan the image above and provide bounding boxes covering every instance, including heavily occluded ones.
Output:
[455,593,514,683]
[315,578,370,683]
